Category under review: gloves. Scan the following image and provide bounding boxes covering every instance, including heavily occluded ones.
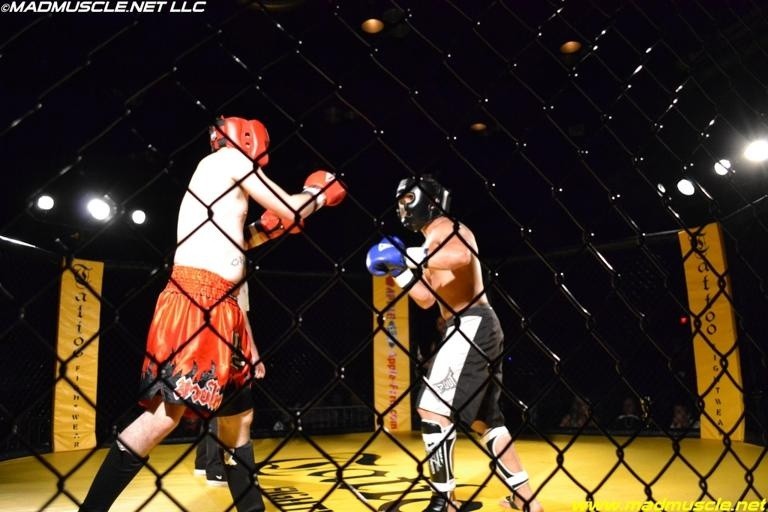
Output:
[301,169,349,211]
[245,209,305,249]
[379,235,416,289]
[365,242,429,279]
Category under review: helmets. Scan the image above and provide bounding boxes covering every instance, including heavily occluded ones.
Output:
[394,176,453,234]
[209,115,272,168]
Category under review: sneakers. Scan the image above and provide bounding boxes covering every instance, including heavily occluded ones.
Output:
[204,469,232,488]
[194,464,207,476]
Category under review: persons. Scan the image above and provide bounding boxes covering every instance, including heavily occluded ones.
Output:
[365,175,546,512]
[76,116,351,512]
[193,417,228,485]
[657,400,700,437]
[558,389,600,429]
[606,394,651,436]
